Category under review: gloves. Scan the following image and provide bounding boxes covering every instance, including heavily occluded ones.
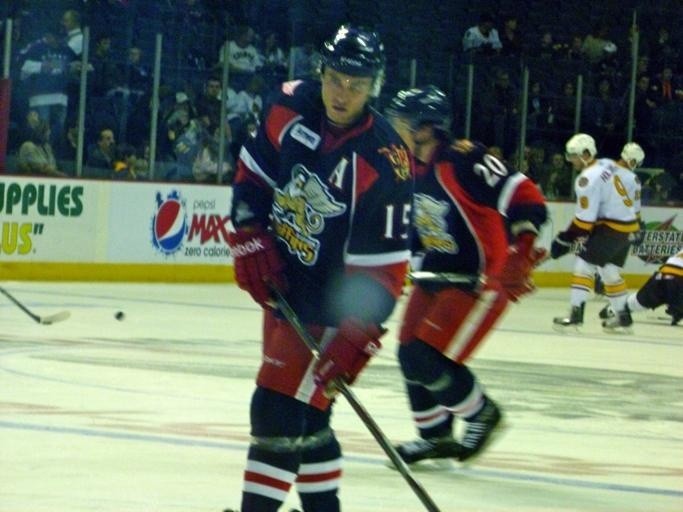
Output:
[550,231,572,259]
[228,223,288,311]
[498,252,534,302]
[313,317,387,399]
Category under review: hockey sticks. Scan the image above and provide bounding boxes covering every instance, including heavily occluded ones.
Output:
[2,284,72,325]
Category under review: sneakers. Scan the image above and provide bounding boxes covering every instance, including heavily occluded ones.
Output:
[553,303,631,328]
[394,415,459,465]
[457,393,499,460]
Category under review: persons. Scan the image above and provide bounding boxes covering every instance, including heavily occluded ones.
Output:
[14,11,319,183]
[546,134,643,330]
[214,26,416,512]
[383,83,546,467]
[462,14,682,201]
[599,248,683,327]
[592,140,645,315]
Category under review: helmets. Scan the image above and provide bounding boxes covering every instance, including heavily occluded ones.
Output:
[383,84,453,133]
[565,134,597,156]
[620,141,645,164]
[316,23,385,86]
